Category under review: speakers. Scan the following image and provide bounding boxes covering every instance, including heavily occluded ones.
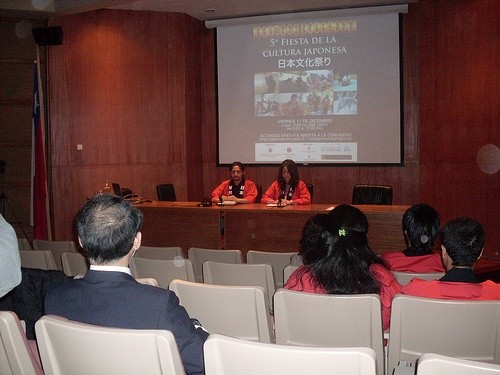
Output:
[31,26,63,46]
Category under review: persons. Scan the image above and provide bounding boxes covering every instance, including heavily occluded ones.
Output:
[210,161,258,230]
[0,214,21,299]
[398,217,500,300]
[336,91,357,113]
[306,94,331,116]
[261,159,311,204]
[290,68,332,86]
[256,98,279,116]
[343,74,351,84]
[282,213,403,346]
[45,194,210,375]
[330,203,369,232]
[380,203,446,273]
[283,94,305,116]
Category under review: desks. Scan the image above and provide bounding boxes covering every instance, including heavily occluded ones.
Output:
[127,200,412,264]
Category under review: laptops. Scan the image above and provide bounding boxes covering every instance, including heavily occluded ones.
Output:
[111,183,124,198]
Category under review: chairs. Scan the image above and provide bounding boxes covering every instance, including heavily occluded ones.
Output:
[157,183,177,202]
[0,237,500,375]
[352,184,393,205]
